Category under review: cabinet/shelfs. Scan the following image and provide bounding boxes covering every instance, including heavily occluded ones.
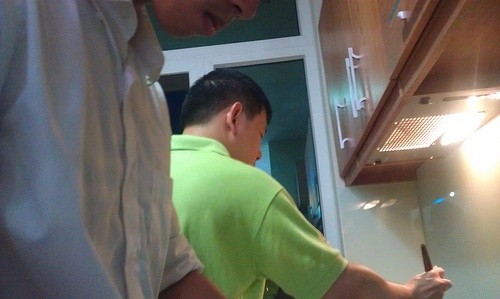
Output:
[318,0,500,187]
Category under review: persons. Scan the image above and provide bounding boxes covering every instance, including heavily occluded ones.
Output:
[168,68,453,299]
[0,0,271,298]
